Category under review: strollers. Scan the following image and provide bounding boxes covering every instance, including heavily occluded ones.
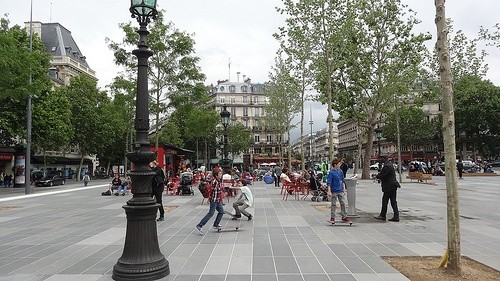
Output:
[179,171,194,196]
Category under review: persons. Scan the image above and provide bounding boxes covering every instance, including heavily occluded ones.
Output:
[414,162,418,170]
[301,169,311,182]
[272,164,281,187]
[83,173,90,186]
[484,165,494,173]
[150,160,165,221]
[30,173,43,185]
[431,166,443,176]
[111,173,132,196]
[457,159,463,178]
[310,172,327,195]
[0,172,13,188]
[340,158,348,178]
[196,168,225,233]
[168,162,173,177]
[223,166,250,195]
[178,163,191,172]
[228,180,254,221]
[374,154,400,222]
[280,169,295,195]
[326,158,351,222]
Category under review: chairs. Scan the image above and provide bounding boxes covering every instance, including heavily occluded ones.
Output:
[166,172,255,205]
[280,177,313,201]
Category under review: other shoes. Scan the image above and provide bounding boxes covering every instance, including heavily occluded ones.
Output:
[374,214,386,220]
[248,214,253,221]
[234,214,241,218]
[214,225,223,229]
[330,217,336,221]
[388,217,399,221]
[195,225,203,234]
[342,216,351,221]
[121,193,125,196]
[157,217,164,221]
[114,193,119,196]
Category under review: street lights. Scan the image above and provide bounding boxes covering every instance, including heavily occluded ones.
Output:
[220,141,224,160]
[219,104,232,159]
[373,125,382,172]
[112,0,171,281]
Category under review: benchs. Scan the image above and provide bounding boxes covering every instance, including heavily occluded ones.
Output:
[406,171,433,184]
[109,180,132,196]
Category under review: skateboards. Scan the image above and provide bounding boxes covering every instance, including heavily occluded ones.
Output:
[214,225,239,232]
[326,220,353,227]
[223,210,237,220]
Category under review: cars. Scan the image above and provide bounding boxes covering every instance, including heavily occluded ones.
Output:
[370,161,384,170]
[35,174,66,188]
[408,160,481,176]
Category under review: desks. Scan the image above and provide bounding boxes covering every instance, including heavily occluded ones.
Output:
[223,183,233,203]
